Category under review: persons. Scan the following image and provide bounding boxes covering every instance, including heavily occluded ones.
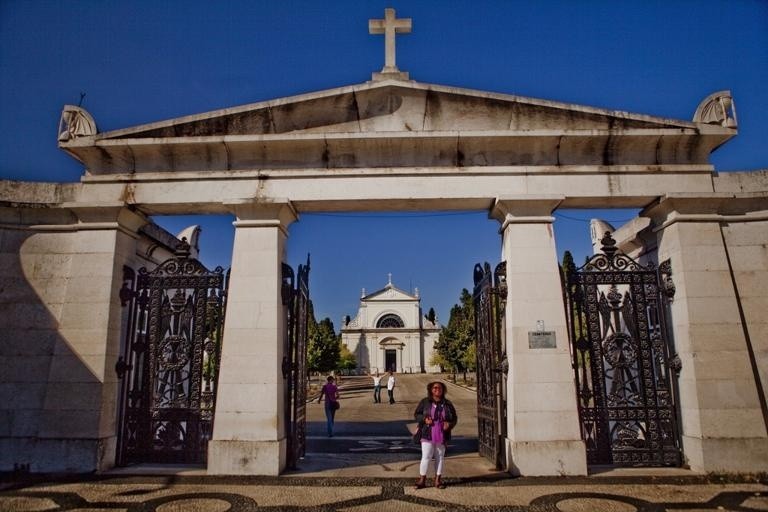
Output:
[387,371,396,404]
[318,376,339,437]
[361,369,388,403]
[414,381,458,489]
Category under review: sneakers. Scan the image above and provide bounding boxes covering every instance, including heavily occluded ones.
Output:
[434,483,445,489]
[414,483,425,489]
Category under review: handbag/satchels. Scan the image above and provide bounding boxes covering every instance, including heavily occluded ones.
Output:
[414,421,421,446]
[329,400,340,409]
